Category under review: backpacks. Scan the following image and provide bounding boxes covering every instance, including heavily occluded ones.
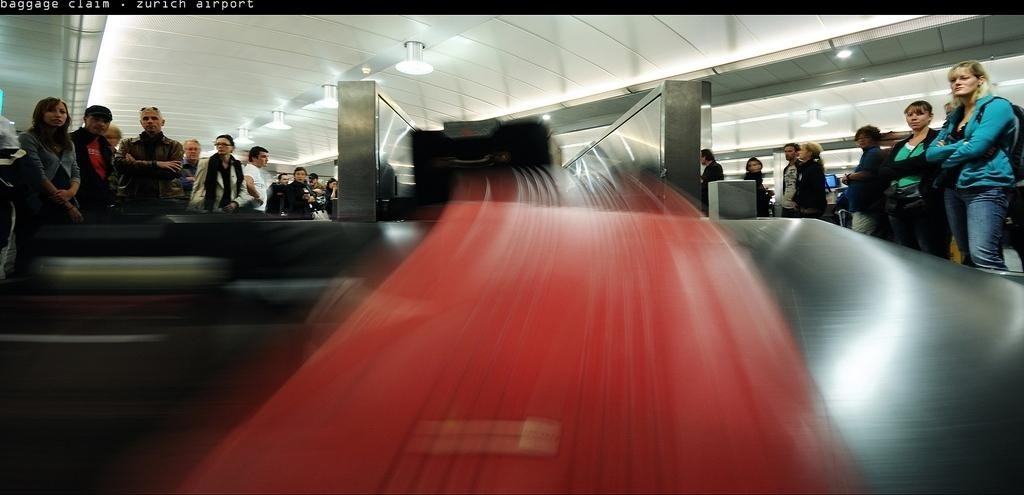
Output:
[974,96,1023,185]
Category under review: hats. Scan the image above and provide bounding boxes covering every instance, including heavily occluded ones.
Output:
[308,173,319,180]
[83,105,113,121]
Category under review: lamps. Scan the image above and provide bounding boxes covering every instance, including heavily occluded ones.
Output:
[315,85,339,107]
[233,128,255,143]
[800,109,829,128]
[395,41,434,75]
[265,110,292,130]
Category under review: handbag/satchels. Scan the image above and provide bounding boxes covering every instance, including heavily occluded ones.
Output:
[881,182,923,215]
[309,207,330,221]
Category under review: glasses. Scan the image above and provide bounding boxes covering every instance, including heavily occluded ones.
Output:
[214,142,232,148]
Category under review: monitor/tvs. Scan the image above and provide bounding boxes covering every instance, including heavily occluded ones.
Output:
[825,174,837,188]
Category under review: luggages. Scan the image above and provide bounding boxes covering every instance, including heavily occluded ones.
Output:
[409,117,565,169]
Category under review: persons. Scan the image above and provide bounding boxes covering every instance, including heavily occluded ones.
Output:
[794,142,826,222]
[700,148,723,207]
[780,141,800,219]
[744,157,775,217]
[0,96,340,278]
[839,59,1022,280]
